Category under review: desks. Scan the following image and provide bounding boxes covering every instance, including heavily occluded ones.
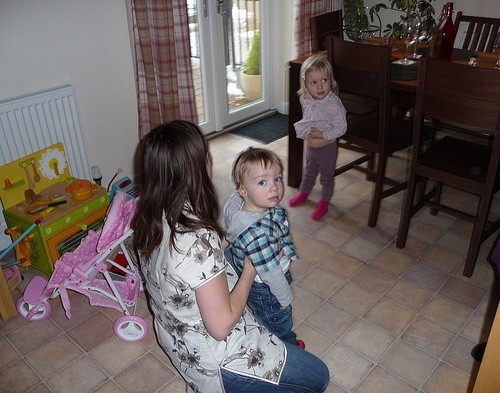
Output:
[288,36,500,190]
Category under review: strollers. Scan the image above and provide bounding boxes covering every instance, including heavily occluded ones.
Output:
[16,189,147,342]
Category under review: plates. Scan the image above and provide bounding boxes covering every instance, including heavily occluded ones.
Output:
[24,202,58,218]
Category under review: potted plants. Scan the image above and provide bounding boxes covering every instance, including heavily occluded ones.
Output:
[239,32,261,102]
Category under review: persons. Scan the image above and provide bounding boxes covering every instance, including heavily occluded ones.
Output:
[222,147,306,354]
[132,119,332,391]
[289,56,348,222]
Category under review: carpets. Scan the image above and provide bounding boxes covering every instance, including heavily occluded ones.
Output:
[229,113,289,144]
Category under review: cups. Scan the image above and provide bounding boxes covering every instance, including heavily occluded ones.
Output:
[67,180,92,201]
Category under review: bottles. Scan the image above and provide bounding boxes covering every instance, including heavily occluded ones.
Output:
[438,2,455,62]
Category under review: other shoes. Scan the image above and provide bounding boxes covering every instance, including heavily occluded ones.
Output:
[295,340,305,350]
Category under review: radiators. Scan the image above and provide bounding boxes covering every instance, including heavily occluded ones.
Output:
[0,85,102,252]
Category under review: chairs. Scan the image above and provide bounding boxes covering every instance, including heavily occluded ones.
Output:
[453,11,500,52]
[324,33,436,228]
[0,262,22,321]
[309,8,376,183]
[396,56,500,279]
